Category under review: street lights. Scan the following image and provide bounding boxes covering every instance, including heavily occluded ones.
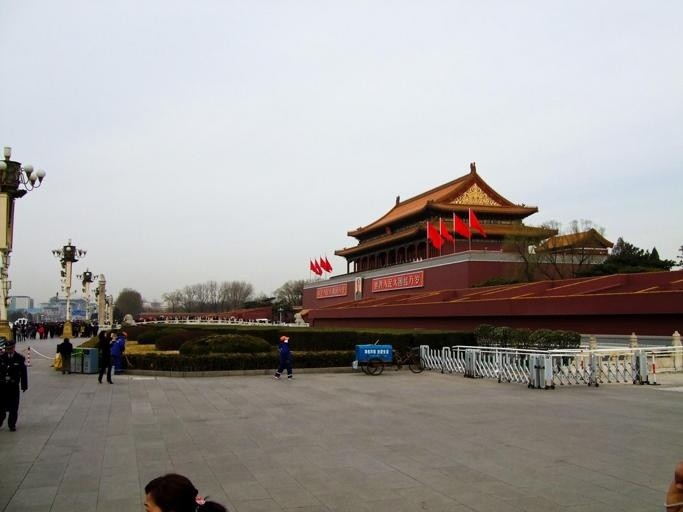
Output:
[50,237,99,339]
[0,145,45,342]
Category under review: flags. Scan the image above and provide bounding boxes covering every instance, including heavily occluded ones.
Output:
[469,208,486,237]
[453,214,472,239]
[427,222,445,249]
[440,217,454,242]
[310,257,332,276]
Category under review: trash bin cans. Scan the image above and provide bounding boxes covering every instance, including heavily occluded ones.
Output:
[70,347,98,373]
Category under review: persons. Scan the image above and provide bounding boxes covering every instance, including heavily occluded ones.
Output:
[144,473,229,511]
[12,317,127,384]
[272,336,296,380]
[0,340,28,431]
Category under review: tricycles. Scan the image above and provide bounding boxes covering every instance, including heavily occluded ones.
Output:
[353,338,426,377]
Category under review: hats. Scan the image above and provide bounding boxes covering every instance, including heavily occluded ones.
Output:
[280,336,289,341]
[3,341,15,349]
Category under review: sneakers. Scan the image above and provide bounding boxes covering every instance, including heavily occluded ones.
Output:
[273,376,295,380]
[98,379,113,385]
[9,426,16,432]
